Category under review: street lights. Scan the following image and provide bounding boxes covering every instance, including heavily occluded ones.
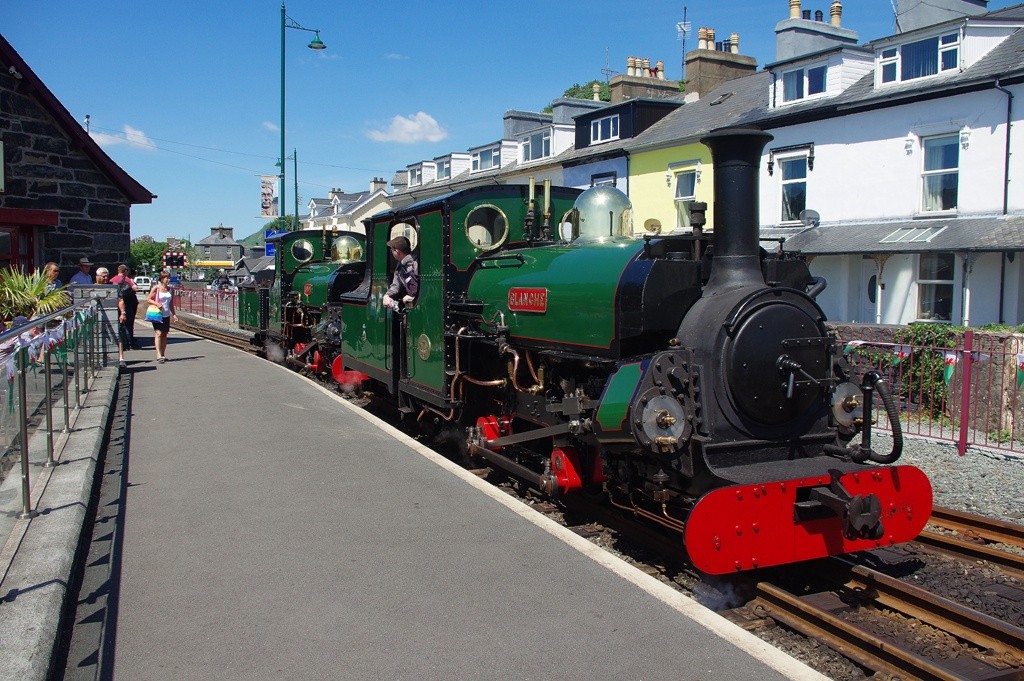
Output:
[280,0,327,215]
[275,148,298,231]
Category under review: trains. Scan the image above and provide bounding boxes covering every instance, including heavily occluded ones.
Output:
[237,174,935,576]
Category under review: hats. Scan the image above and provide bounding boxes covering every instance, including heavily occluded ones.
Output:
[79,258,94,266]
[386,236,411,251]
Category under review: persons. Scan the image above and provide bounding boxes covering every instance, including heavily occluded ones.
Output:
[217,269,233,292]
[261,180,278,216]
[147,271,178,363]
[93,267,129,371]
[39,262,63,296]
[66,257,94,300]
[0,311,45,366]
[109,264,139,350]
[382,236,420,309]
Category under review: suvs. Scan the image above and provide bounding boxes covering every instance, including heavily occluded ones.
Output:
[135,277,151,294]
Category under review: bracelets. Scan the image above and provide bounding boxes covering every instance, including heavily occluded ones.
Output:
[121,311,126,315]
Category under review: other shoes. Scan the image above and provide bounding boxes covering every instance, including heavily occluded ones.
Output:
[118,359,126,369]
[156,354,166,364]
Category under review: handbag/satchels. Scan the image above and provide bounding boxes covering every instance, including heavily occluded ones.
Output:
[144,303,164,323]
[118,276,139,307]
